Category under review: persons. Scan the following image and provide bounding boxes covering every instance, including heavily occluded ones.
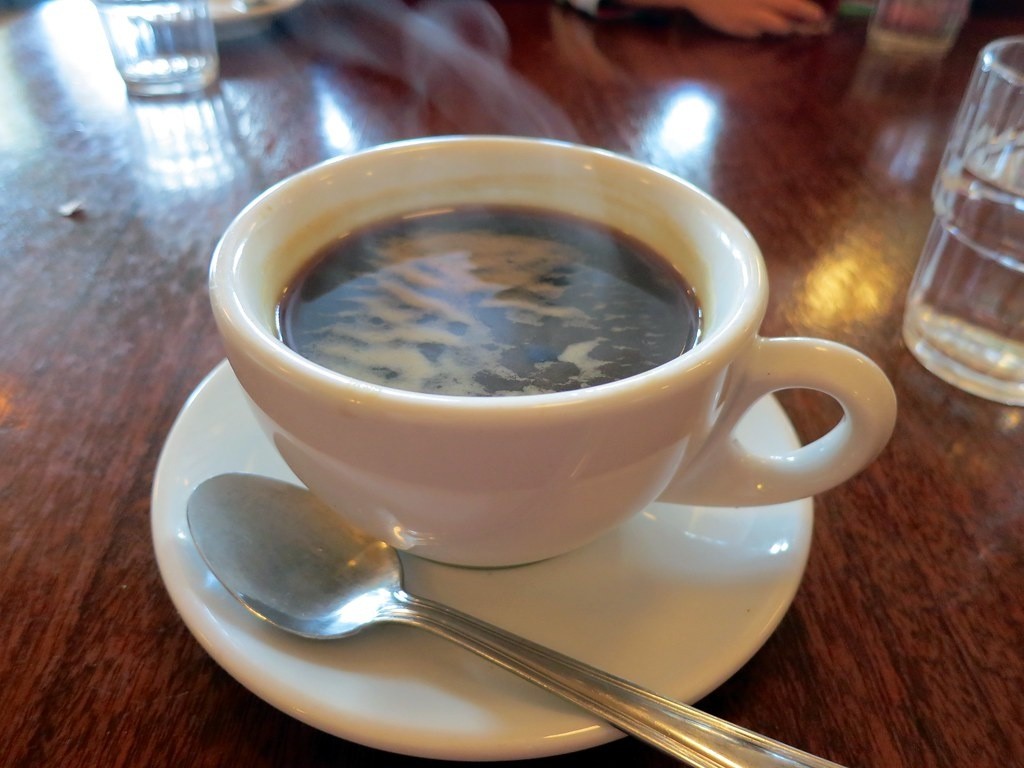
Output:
[569,0,824,37]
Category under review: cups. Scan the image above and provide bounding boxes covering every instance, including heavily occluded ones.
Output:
[90,0,220,96]
[902,34,1024,407]
[864,0,971,64]
[208,134,897,567]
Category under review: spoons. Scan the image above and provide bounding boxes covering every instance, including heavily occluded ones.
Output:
[188,473,852,768]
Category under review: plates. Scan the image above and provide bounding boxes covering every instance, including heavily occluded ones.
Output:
[213,0,306,44]
[149,350,819,762]
